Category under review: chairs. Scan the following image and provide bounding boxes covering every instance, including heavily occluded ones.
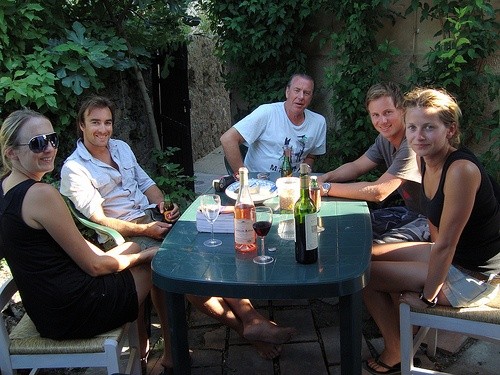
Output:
[400,274,500,375]
[0,255,143,375]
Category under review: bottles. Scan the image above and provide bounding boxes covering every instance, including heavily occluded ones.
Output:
[276,177,303,210]
[234,166,259,255]
[293,164,319,265]
[310,176,322,230]
[162,194,179,228]
[281,157,293,177]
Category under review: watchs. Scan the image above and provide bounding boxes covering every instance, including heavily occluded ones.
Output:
[323,183,331,197]
[419,292,438,308]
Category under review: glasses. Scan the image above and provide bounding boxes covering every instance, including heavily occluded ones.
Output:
[15,132,60,154]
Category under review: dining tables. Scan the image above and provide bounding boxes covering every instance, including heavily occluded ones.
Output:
[151,173,373,375]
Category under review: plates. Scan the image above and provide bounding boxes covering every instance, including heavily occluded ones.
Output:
[225,179,278,205]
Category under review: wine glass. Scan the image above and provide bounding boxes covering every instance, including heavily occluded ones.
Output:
[199,193,223,247]
[250,206,275,265]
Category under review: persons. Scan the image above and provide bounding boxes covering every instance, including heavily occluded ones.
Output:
[360,89,500,375]
[60,96,297,360]
[306,80,431,244]
[220,71,326,180]
[0,109,190,368]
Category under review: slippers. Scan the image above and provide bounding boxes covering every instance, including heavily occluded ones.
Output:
[365,357,400,375]
